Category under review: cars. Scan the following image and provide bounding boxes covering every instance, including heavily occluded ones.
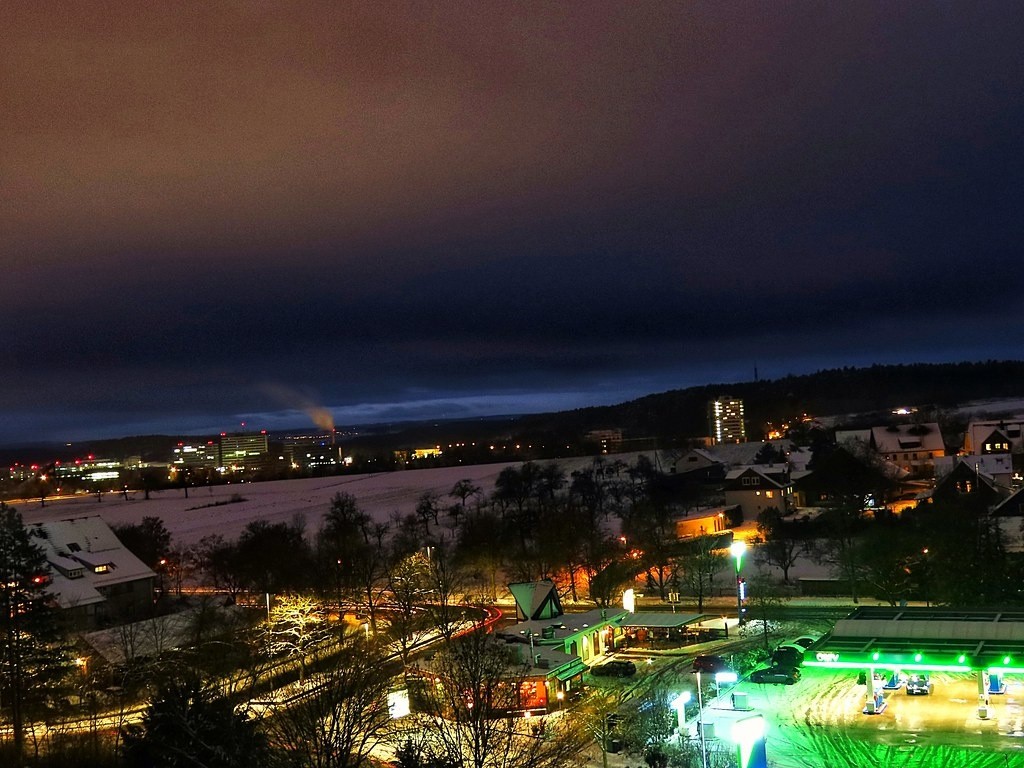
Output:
[57,686,131,713]
[693,654,727,674]
[770,635,822,666]
[186,639,235,658]
[750,665,803,685]
[591,660,636,679]
[905,675,930,695]
